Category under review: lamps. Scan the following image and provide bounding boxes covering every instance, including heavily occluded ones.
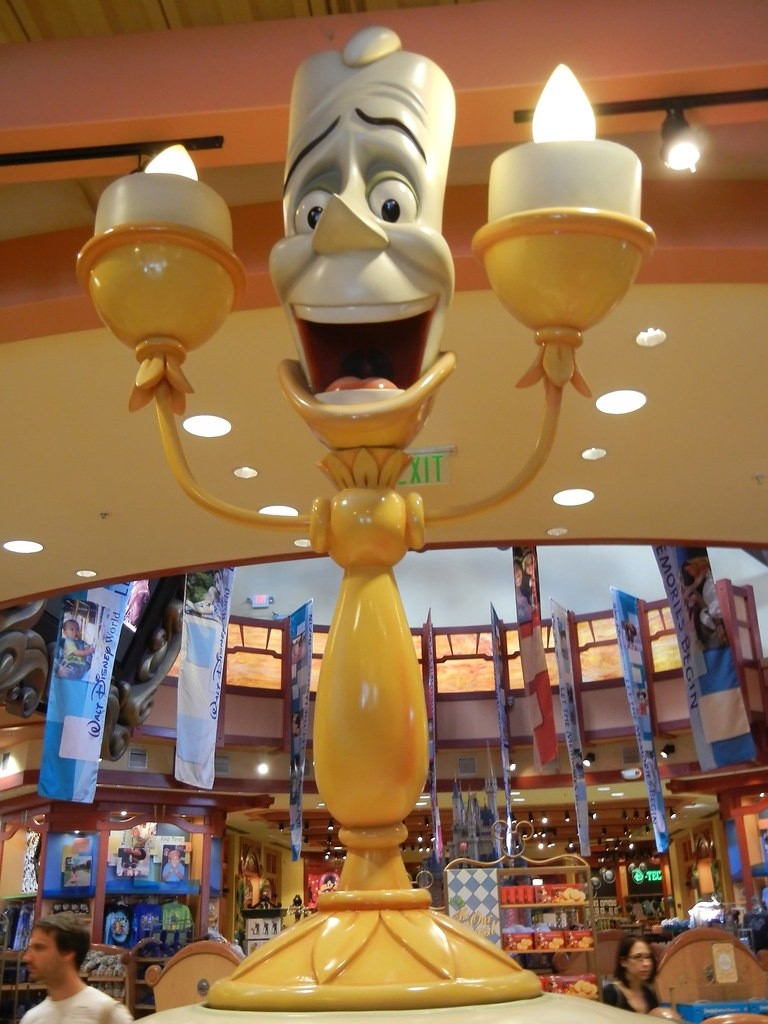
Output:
[510,807,677,863]
[426,845,430,852]
[332,850,339,861]
[402,846,407,851]
[417,832,423,843]
[484,63,644,218]
[411,845,415,852]
[430,834,433,841]
[418,845,423,852]
[326,834,332,844]
[94,144,235,251]
[424,815,430,826]
[279,822,285,831]
[659,744,675,759]
[583,752,595,767]
[304,835,308,843]
[327,818,335,831]
[323,845,331,859]
[342,852,346,859]
[510,760,517,772]
[304,822,310,831]
[658,107,701,172]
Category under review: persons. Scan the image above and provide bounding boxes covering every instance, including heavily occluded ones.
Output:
[19,913,138,1024]
[56,619,97,678]
[513,549,539,622]
[599,934,665,1010]
[162,850,186,883]
[184,569,228,623]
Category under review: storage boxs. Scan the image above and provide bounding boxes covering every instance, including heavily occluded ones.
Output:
[534,931,565,949]
[500,885,508,905]
[585,899,618,922]
[502,932,533,950]
[508,886,517,904]
[517,886,525,904]
[510,954,525,969]
[552,883,584,903]
[565,930,594,948]
[543,884,552,904]
[525,953,552,969]
[550,972,600,1002]
[525,885,535,904]
[534,885,543,904]
[538,974,551,992]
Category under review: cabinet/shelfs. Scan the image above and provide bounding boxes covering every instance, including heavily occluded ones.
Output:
[444,853,605,1004]
[240,895,289,956]
[0,937,175,1024]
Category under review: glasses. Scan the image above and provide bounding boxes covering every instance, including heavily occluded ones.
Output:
[630,953,653,963]
